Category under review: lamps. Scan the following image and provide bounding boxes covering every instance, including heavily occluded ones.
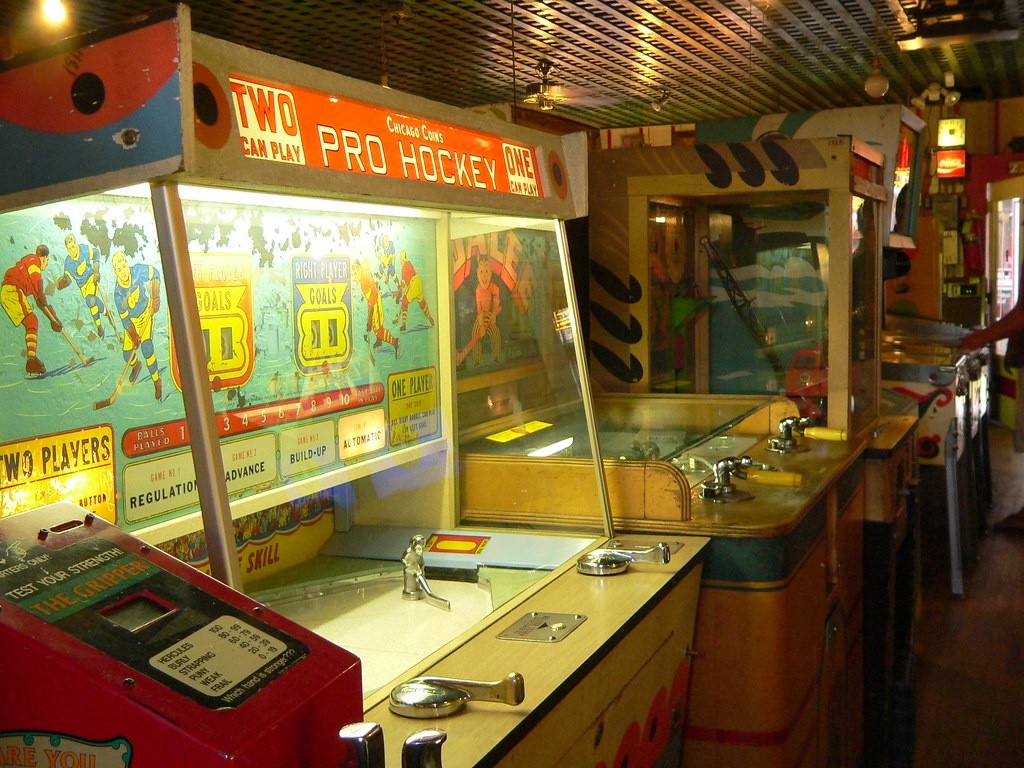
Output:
[652,89,671,112]
[378,11,413,89]
[911,82,961,110]
[523,62,575,110]
[864,10,889,96]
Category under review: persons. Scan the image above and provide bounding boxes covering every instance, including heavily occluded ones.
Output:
[852,204,875,414]
[957,218,1024,532]
